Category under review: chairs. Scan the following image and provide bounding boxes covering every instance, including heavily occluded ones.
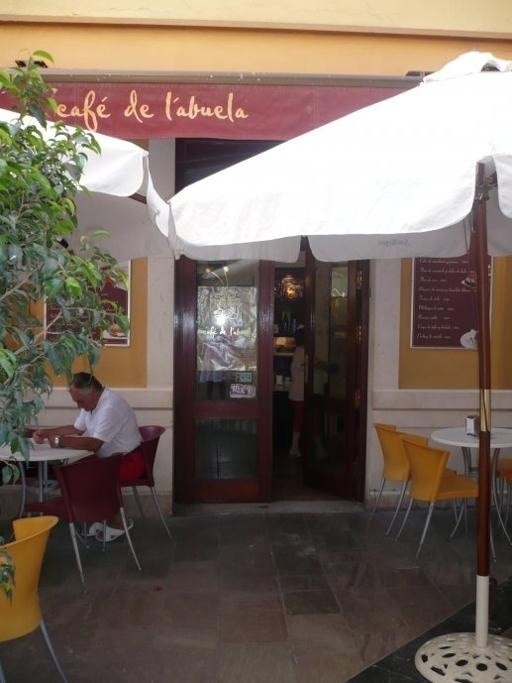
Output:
[2,516,68,682]
[369,421,512,564]
[46,425,175,593]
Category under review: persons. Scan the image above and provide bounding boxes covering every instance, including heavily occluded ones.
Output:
[32,371,146,543]
[286,326,314,462]
[204,315,252,397]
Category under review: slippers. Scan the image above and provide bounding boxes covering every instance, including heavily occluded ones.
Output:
[81,517,134,543]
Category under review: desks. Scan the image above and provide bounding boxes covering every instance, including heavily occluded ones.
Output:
[1,445,89,521]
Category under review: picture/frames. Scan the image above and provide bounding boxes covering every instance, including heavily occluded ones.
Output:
[408,256,494,350]
[41,259,131,349]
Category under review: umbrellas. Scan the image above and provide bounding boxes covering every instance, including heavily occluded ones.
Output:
[0,104,177,272]
[168,47,511,650]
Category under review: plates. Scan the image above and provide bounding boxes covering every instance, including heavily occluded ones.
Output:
[460,331,480,350]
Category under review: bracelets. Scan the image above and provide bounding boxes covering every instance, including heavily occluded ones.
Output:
[54,432,64,448]
